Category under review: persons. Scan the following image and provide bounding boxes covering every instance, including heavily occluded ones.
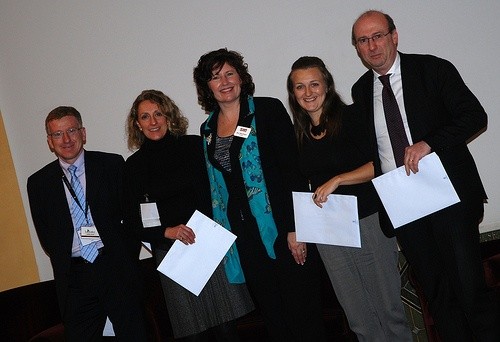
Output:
[352,10,500,342]
[193,47,328,342]
[287,56,415,342]
[26,106,148,342]
[126,89,256,342]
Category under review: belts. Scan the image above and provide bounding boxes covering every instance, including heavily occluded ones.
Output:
[69,248,103,264]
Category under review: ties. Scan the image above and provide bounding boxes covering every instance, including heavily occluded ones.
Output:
[68,165,99,264]
[378,73,411,168]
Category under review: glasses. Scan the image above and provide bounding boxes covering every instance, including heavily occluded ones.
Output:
[354,32,390,47]
[49,127,83,138]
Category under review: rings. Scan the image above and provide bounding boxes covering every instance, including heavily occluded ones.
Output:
[179,237,182,241]
[409,159,414,162]
[312,194,317,199]
[301,248,305,254]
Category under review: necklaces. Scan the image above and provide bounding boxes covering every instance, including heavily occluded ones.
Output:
[311,121,325,137]
